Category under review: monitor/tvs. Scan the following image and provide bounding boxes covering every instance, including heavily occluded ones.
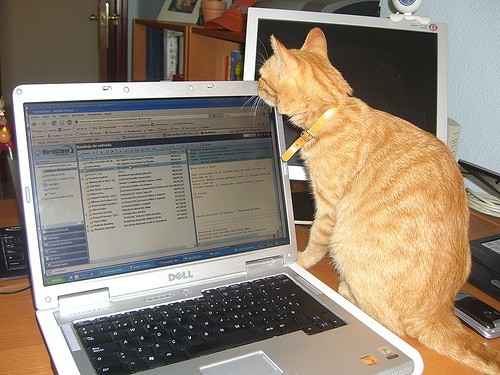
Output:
[243,7,449,226]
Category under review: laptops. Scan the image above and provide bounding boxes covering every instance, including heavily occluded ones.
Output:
[10,80,425,375]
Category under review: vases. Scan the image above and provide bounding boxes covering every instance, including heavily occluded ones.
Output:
[201,0,227,27]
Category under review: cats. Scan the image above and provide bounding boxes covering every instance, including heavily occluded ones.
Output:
[241,27,500,374]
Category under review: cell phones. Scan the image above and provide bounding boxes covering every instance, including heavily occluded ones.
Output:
[453,291,500,339]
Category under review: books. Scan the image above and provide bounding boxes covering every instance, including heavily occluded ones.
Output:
[291,192,316,226]
[145,25,184,83]
[231,50,244,81]
[225,56,229,81]
[229,57,231,81]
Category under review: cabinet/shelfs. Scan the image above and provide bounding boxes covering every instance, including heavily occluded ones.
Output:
[129,18,246,82]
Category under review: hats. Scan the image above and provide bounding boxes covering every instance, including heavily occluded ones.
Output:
[204,0,256,36]
